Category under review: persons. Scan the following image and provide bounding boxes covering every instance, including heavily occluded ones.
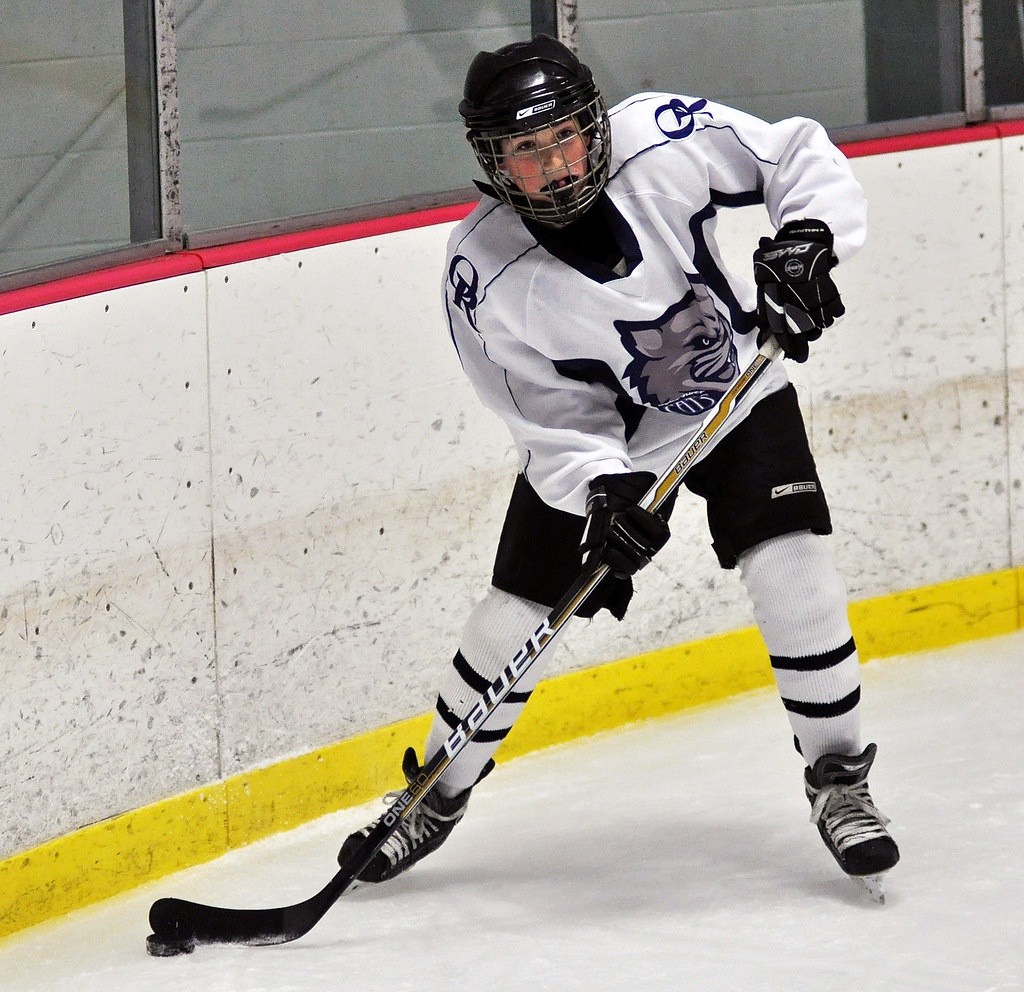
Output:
[338,39,902,902]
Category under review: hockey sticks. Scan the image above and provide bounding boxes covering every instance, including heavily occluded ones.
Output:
[148,327,784,949]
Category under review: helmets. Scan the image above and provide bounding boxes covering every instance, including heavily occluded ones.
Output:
[458,33,612,227]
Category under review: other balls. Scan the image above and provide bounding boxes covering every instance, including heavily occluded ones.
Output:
[145,933,195,958]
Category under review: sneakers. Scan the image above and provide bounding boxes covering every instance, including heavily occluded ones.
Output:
[337,746,496,897]
[793,734,900,910]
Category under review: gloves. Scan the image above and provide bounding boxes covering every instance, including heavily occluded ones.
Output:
[753,218,846,363]
[575,470,671,580]
